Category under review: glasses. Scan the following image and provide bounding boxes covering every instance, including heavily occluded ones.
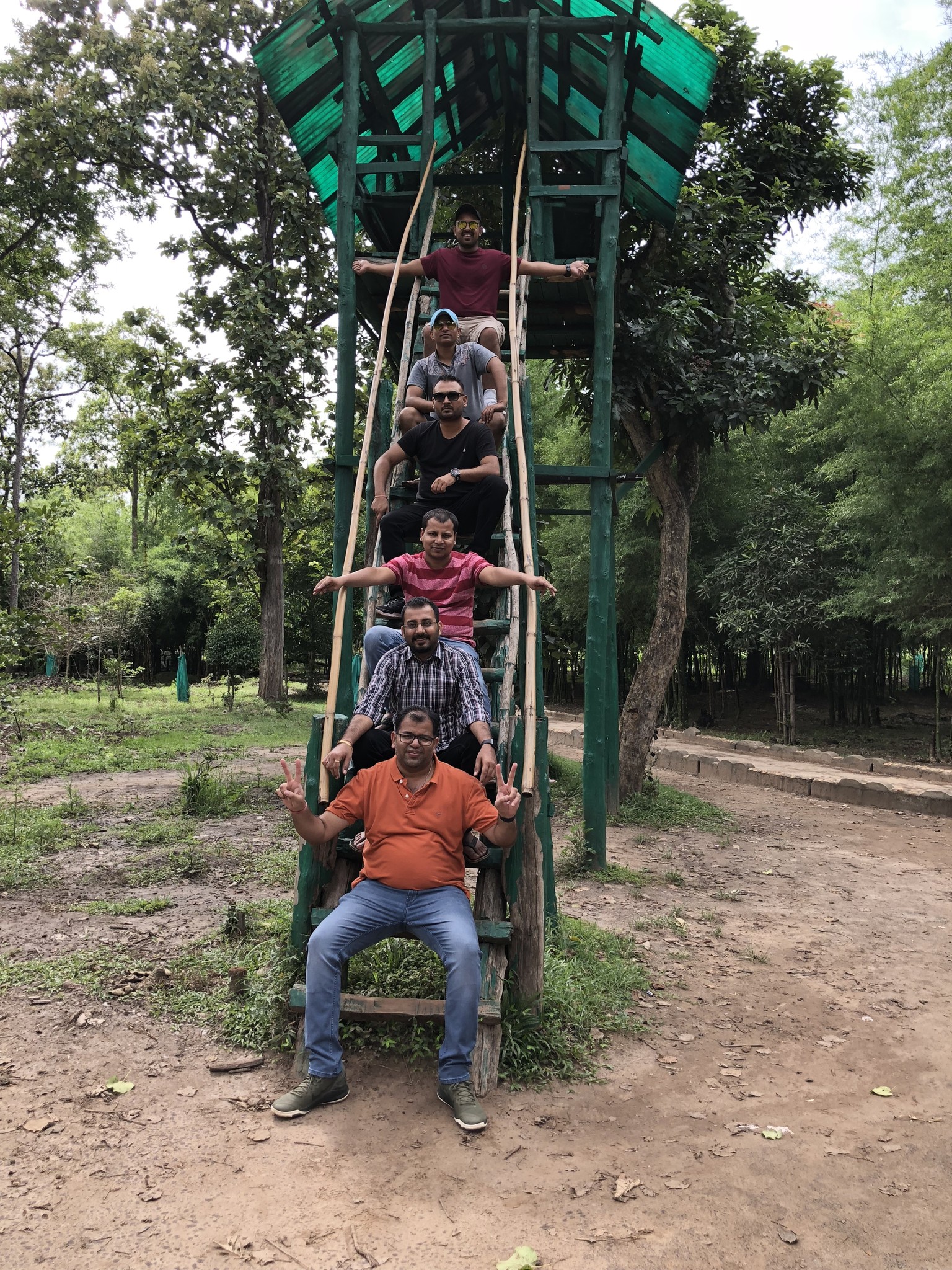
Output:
[432,320,458,331]
[455,220,482,230]
[433,392,465,402]
[395,732,435,746]
[403,621,438,629]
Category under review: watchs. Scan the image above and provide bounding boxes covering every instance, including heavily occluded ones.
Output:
[450,468,461,483]
[564,262,573,279]
[479,738,498,751]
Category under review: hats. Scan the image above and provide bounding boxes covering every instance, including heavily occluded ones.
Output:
[430,308,460,332]
[454,202,484,224]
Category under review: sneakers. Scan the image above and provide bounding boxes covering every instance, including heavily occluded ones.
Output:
[436,1077,488,1131]
[271,1061,350,1118]
[375,595,406,619]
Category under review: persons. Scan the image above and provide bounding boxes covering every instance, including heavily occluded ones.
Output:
[352,202,589,421]
[398,308,508,489]
[313,509,559,731]
[370,373,509,620]
[270,704,523,1130]
[321,597,502,866]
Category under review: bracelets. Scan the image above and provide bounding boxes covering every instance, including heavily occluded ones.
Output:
[335,739,354,753]
[498,811,517,823]
[285,800,307,815]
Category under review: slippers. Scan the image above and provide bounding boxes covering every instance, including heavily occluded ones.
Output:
[401,476,420,490]
[462,835,490,864]
[348,836,367,852]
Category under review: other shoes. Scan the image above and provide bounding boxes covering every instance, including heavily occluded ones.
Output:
[484,395,507,416]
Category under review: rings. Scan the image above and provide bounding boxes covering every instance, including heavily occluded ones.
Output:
[325,760,330,765]
[333,759,340,764]
